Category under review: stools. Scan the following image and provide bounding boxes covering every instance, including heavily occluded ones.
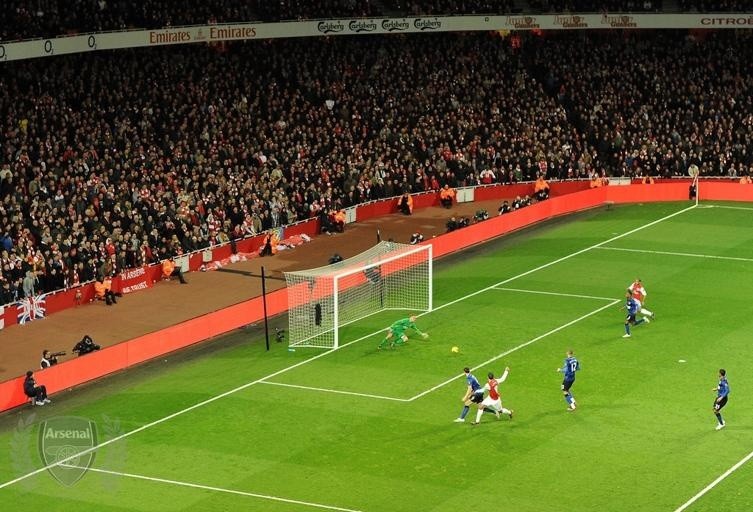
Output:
[26,397,36,406]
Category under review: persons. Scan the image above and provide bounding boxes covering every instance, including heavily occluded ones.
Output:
[618,288,650,339]
[39,349,59,371]
[469,365,514,427]
[22,370,51,406]
[625,276,654,321]
[452,366,502,424]
[711,368,730,432]
[70,335,104,358]
[555,348,580,412]
[376,313,428,351]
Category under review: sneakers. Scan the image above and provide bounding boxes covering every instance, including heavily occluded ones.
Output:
[453,417,465,423]
[470,421,480,425]
[716,420,726,430]
[495,410,514,420]
[643,312,655,323]
[622,333,631,338]
[567,402,577,411]
[35,398,51,406]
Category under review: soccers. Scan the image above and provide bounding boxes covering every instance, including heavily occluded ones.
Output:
[451,346,459,354]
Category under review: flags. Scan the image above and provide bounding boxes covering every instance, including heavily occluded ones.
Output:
[13,294,48,325]
